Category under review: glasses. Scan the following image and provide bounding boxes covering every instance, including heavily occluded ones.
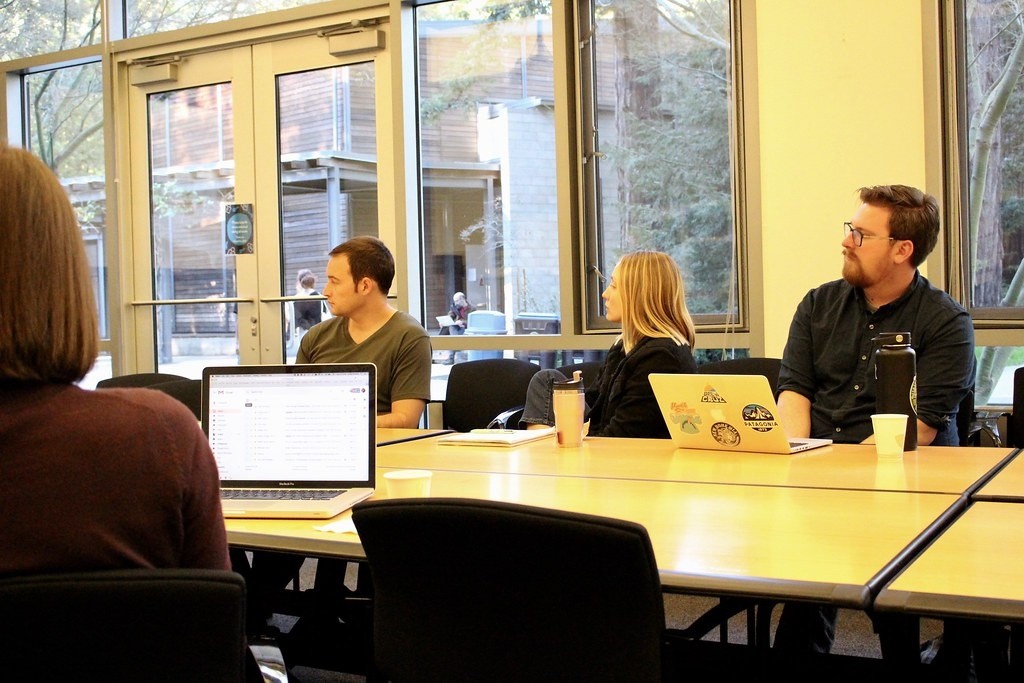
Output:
[844,222,898,246]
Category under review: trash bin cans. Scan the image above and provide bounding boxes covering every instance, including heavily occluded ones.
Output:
[463,311,508,361]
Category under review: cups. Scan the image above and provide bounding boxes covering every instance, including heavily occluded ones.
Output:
[871,414,909,457]
[553,370,585,448]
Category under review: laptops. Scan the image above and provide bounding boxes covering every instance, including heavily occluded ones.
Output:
[648,373,833,454]
[200,361,378,520]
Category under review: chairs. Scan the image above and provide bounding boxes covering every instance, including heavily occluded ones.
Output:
[0,356,1024,683]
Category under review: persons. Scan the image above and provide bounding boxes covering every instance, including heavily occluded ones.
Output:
[297,236,432,428]
[289,268,333,334]
[442,292,477,365]
[518,249,696,440]
[0,142,235,576]
[774,184,977,661]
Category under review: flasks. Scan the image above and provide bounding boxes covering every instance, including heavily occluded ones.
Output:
[871,332,917,452]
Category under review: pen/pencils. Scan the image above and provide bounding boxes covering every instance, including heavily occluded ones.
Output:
[470,429,512,434]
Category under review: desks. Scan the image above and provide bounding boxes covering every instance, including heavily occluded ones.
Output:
[376,425,456,448]
[375,427,1017,497]
[875,501,1024,683]
[222,464,960,683]
[968,446,1024,503]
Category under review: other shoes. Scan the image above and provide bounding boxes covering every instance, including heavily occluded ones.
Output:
[442,357,453,365]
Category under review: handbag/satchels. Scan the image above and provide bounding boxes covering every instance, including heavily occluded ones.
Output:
[318,292,333,322]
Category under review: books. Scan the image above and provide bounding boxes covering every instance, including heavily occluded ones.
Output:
[437,426,557,447]
